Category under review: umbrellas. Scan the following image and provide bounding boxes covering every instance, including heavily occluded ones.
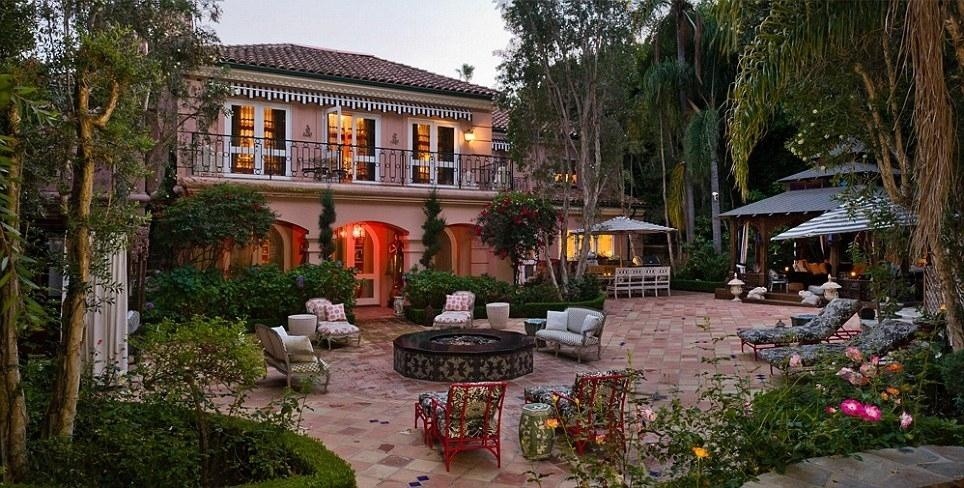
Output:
[569,214,680,267]
[770,188,923,325]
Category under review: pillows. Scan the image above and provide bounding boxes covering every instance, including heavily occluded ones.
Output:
[546,310,569,332]
[580,314,599,337]
[283,335,318,362]
[792,259,828,276]
[445,294,470,311]
[271,325,288,339]
[324,303,347,321]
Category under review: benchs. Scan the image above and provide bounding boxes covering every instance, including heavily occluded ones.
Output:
[607,266,671,300]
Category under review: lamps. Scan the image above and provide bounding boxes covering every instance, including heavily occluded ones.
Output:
[464,129,473,142]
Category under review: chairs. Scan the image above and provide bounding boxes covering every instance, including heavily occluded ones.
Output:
[758,319,917,370]
[737,297,862,361]
[305,298,361,351]
[523,368,629,457]
[768,269,789,293]
[414,381,506,472]
[433,291,475,330]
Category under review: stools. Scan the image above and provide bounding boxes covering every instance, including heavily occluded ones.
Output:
[519,403,554,460]
[288,313,317,343]
[486,301,510,328]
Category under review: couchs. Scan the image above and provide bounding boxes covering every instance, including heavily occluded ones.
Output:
[254,321,330,394]
[535,305,607,363]
